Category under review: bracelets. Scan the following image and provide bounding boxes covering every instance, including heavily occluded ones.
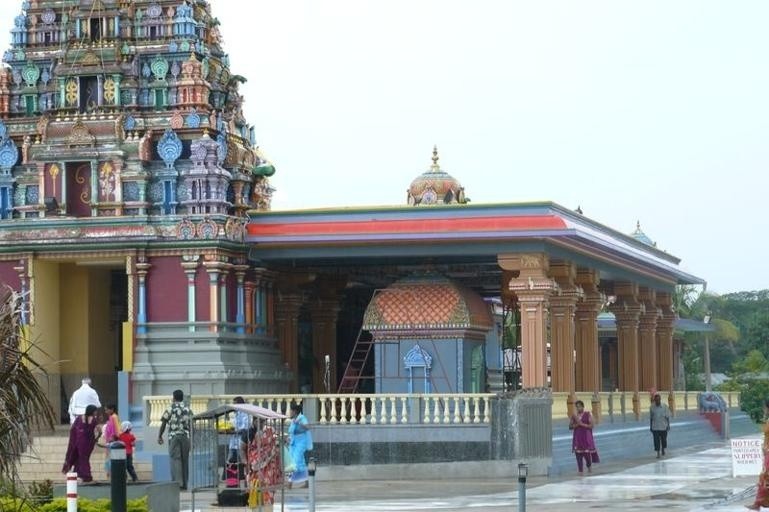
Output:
[584,424,588,428]
[298,421,301,425]
[572,424,577,429]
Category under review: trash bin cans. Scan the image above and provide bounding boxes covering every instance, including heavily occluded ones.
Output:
[144,482,180,512]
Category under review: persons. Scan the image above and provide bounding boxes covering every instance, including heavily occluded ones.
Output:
[240,416,291,512]
[744,400,769,512]
[62,402,139,482]
[220,396,255,488]
[649,394,670,459]
[68,377,101,427]
[569,401,600,475]
[285,403,313,490]
[158,389,194,490]
